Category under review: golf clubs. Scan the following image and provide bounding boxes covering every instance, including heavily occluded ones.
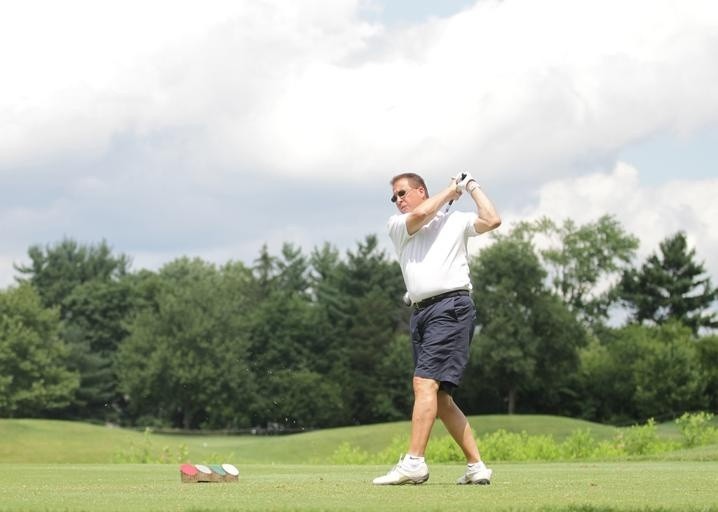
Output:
[401,171,469,307]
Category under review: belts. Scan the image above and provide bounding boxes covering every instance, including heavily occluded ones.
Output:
[414,290,470,310]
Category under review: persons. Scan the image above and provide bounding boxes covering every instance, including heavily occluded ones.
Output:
[371,172,504,486]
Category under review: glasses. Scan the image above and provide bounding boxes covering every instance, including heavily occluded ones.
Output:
[391,186,419,203]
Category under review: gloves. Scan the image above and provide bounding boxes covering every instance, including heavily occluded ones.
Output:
[451,171,476,192]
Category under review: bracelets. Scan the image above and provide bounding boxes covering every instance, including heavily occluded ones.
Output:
[467,181,481,196]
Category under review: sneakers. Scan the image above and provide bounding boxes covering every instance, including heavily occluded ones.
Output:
[455,460,492,484]
[371,451,433,485]
